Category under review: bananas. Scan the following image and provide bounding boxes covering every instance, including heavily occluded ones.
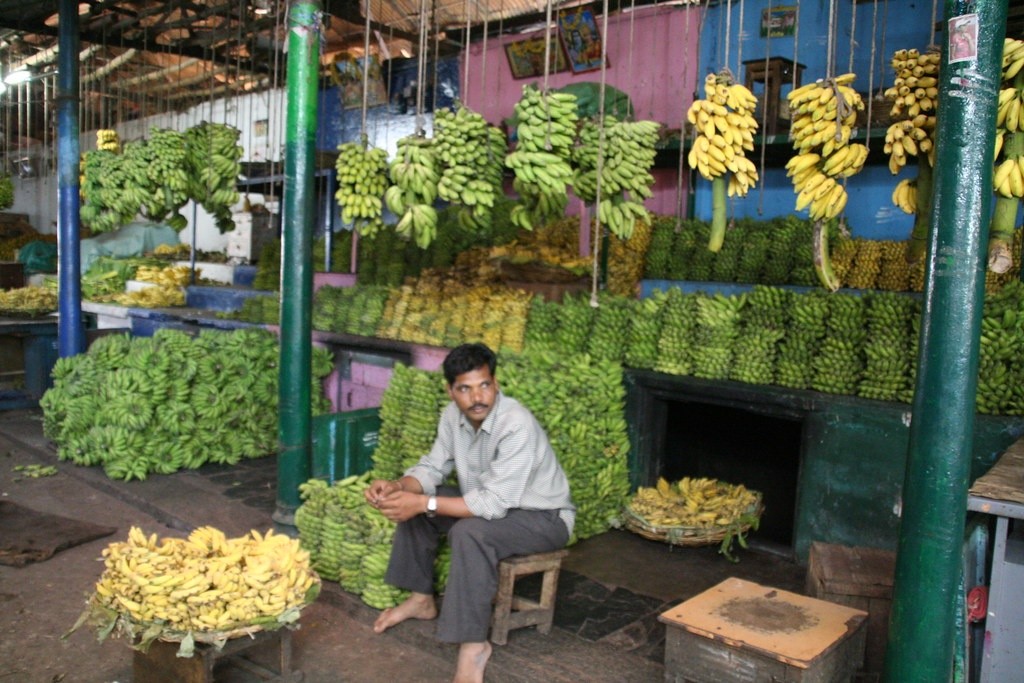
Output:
[0,120,282,482]
[95,525,317,632]
[294,36,1024,614]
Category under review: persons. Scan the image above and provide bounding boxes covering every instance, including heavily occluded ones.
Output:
[363,341,578,683]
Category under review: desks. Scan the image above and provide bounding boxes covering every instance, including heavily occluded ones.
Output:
[967,439,1024,683]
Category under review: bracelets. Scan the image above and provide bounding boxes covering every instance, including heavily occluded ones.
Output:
[392,479,403,490]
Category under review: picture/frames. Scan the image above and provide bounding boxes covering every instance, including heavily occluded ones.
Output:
[555,6,611,74]
[504,34,569,80]
[336,53,389,111]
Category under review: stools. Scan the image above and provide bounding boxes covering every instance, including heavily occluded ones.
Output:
[806,542,896,669]
[132,622,302,683]
[742,56,807,135]
[658,576,870,683]
[489,548,568,646]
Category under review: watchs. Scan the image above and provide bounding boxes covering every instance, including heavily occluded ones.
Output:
[426,495,437,518]
